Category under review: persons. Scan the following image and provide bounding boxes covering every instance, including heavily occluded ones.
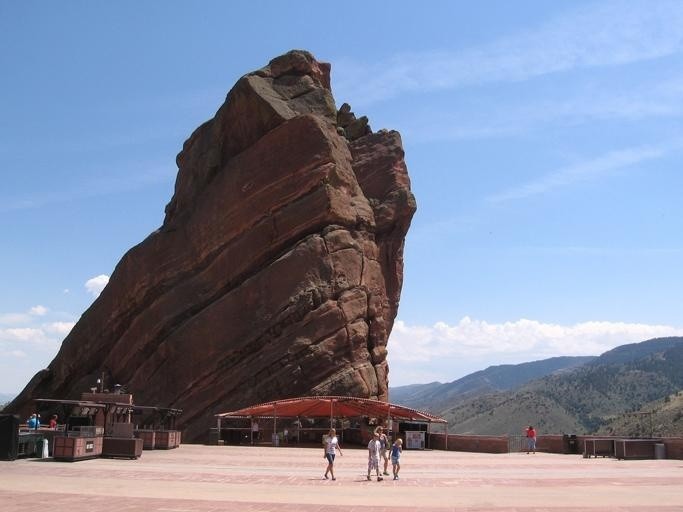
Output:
[366,426,403,481]
[27,414,40,428]
[253,420,259,445]
[526,425,536,454]
[49,415,58,428]
[323,428,342,480]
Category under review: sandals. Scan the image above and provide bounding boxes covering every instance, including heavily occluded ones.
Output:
[324,473,336,480]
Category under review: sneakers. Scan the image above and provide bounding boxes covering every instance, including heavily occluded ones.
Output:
[367,472,400,481]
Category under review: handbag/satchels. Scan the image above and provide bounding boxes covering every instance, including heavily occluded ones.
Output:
[386,442,390,450]
